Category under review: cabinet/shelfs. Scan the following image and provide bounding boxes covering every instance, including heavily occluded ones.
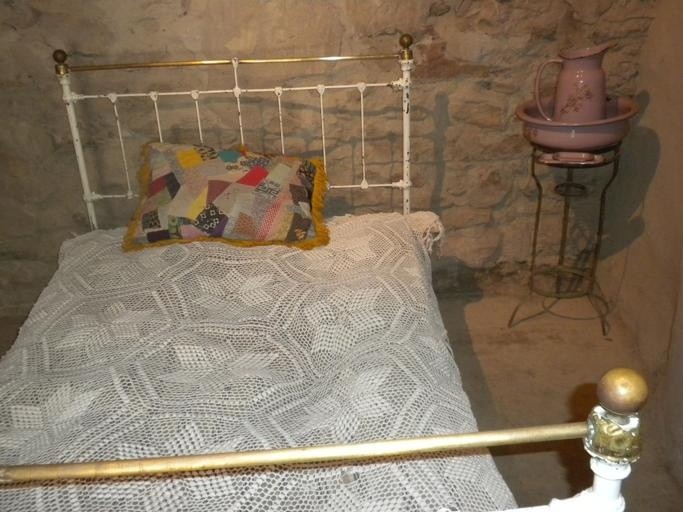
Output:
[508,143,623,339]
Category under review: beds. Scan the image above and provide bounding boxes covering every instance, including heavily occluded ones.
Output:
[0,33,651,512]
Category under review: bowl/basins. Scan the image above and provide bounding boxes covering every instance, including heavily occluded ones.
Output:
[516,94,639,166]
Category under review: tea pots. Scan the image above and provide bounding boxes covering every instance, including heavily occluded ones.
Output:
[535,45,609,122]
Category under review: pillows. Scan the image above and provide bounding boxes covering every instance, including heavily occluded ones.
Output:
[121,141,330,252]
